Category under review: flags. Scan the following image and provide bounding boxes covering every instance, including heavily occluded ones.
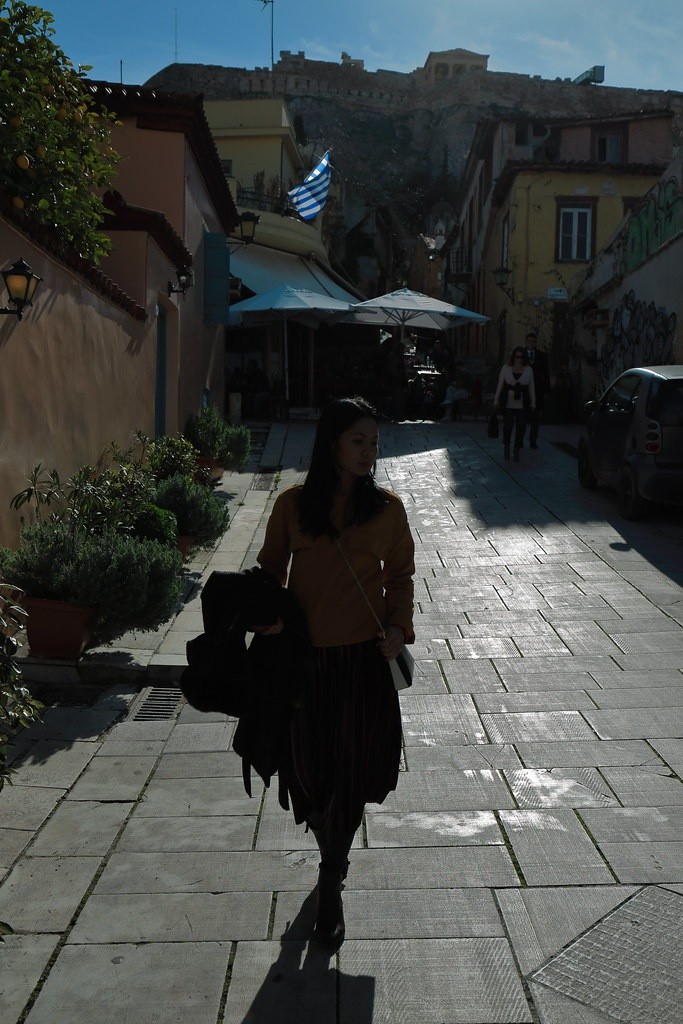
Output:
[285,151,331,223]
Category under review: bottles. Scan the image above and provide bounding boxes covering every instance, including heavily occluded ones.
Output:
[430,361,434,372]
[426,357,430,369]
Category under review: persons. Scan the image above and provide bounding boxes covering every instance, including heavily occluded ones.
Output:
[231,393,414,952]
[522,333,551,449]
[425,337,471,420]
[493,346,536,463]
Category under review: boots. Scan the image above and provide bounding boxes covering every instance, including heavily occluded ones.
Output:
[315,860,348,948]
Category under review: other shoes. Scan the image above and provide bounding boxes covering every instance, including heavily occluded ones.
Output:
[504,447,511,459]
[515,438,524,449]
[528,437,538,449]
[440,400,453,405]
[440,416,451,421]
[513,449,521,462]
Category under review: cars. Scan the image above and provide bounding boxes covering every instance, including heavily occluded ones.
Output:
[576,364,683,522]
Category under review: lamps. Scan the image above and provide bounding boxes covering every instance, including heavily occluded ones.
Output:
[491,263,515,306]
[167,264,195,299]
[225,210,261,254]
[0,256,43,323]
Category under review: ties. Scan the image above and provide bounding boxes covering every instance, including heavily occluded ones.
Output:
[529,351,533,362]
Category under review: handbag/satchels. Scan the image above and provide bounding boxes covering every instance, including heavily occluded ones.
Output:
[379,632,414,692]
[487,408,499,436]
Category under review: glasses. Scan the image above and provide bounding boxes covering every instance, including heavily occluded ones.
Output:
[514,355,524,359]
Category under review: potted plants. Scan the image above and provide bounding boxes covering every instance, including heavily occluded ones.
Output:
[181,409,225,483]
[1,518,149,659]
[152,474,204,561]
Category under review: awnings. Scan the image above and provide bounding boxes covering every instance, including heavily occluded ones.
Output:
[228,238,375,317]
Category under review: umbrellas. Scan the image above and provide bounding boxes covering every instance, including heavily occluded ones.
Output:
[351,287,492,343]
[225,281,356,399]
